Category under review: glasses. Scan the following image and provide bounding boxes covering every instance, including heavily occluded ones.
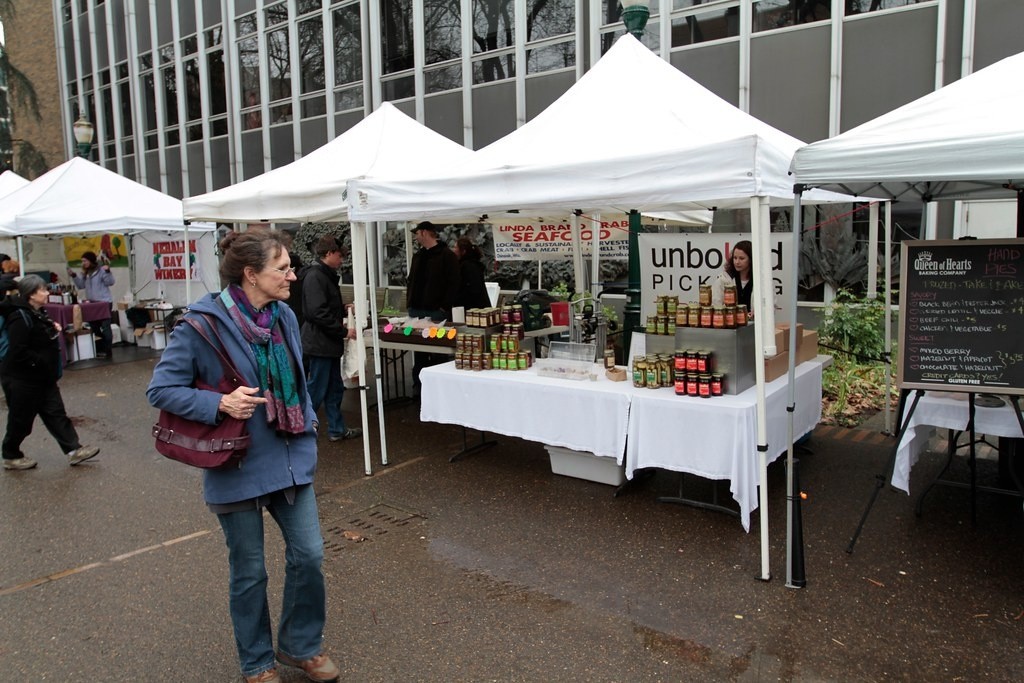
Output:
[263,264,296,279]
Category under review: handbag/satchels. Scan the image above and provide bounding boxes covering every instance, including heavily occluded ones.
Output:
[344,305,368,379]
[152,311,252,471]
[505,289,560,332]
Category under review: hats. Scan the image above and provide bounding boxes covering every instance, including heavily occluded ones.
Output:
[410,221,434,234]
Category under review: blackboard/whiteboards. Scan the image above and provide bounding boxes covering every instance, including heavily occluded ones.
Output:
[897,237,1024,395]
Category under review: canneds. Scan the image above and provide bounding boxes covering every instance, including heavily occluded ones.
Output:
[455,305,533,372]
[645,294,679,336]
[676,285,749,328]
[674,349,724,399]
[632,353,675,390]
[604,349,615,369]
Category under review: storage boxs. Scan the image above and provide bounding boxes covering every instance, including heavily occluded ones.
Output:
[544,445,626,486]
[765,322,818,382]
[73,303,171,362]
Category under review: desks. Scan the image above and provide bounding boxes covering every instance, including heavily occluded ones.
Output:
[906,389,1024,518]
[134,307,186,349]
[421,355,834,519]
[363,325,569,412]
[42,302,109,366]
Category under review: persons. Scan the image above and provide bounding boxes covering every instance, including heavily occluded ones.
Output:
[66,251,115,359]
[0,253,100,469]
[295,235,362,441]
[145,226,338,683]
[406,221,460,322]
[278,231,303,274]
[452,238,492,308]
[718,241,754,321]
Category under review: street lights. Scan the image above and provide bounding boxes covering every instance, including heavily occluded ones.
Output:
[72,109,95,160]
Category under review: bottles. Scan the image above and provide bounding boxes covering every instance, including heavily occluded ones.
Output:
[54,284,77,304]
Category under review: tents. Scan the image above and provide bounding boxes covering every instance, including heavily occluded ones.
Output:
[799,185,895,436]
[784,52,1024,588]
[0,156,220,309]
[0,170,31,199]
[183,100,476,474]
[346,30,814,579]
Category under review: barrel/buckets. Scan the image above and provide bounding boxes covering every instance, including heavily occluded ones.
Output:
[551,302,569,326]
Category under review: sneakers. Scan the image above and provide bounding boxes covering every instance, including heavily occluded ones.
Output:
[242,647,341,683]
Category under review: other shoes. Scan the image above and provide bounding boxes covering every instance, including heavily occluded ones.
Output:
[66,444,100,466]
[2,456,37,470]
[329,427,362,442]
